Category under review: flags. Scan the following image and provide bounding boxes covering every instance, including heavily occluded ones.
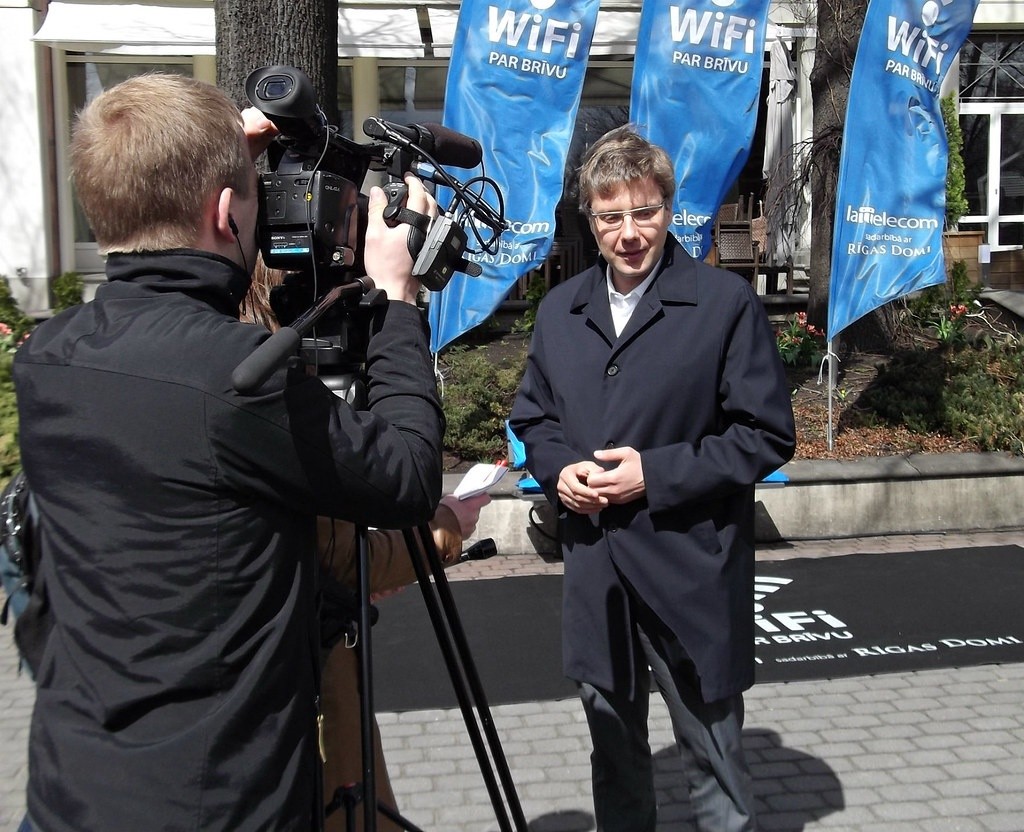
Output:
[827,0,982,339]
[428,0,602,354]
[628,0,771,264]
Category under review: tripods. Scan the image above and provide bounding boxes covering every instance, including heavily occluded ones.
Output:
[233,267,531,832]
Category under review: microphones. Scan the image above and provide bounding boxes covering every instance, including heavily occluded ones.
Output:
[441,538,497,568]
[364,116,483,169]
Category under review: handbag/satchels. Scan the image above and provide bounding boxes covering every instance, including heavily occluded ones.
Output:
[0,469,43,680]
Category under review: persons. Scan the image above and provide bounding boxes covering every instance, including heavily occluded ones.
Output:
[510,123,798,832]
[14,75,491,832]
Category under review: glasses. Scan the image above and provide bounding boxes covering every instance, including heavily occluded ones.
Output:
[589,198,667,228]
